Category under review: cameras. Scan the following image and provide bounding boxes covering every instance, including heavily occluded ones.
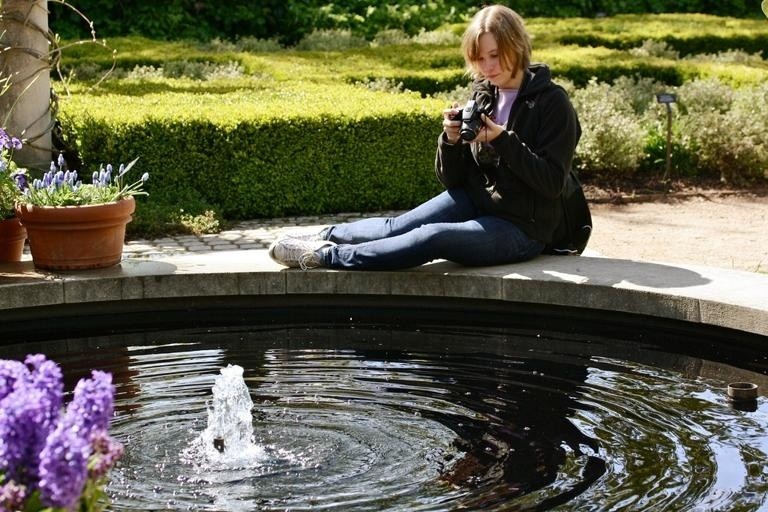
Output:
[448,100,494,140]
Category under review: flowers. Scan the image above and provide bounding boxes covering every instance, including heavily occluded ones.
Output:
[0,128,150,221]
[0,354,123,512]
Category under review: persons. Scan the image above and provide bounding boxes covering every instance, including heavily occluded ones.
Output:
[269,4,592,272]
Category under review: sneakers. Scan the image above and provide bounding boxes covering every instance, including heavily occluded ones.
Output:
[268,225,336,271]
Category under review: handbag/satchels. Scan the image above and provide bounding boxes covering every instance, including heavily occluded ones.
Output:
[553,172,592,255]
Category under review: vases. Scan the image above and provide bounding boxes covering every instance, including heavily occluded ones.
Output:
[15,196,136,270]
[0,217,27,262]
